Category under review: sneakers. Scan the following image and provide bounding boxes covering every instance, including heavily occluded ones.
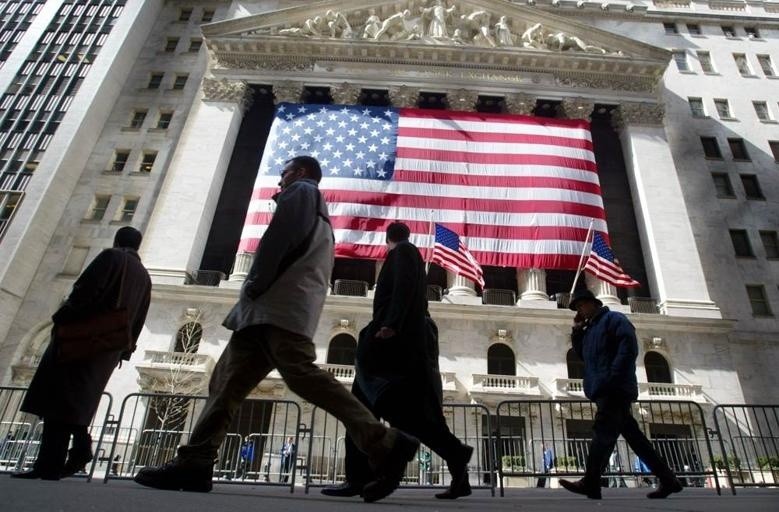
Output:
[435,473,474,501]
[360,429,421,503]
[133,454,216,494]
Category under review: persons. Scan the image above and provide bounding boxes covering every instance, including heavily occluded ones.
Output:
[10,226,152,479]
[415,444,431,487]
[320,223,473,498]
[280,3,606,55]
[559,288,682,499]
[279,433,294,483]
[608,447,622,487]
[391,298,472,499]
[133,157,419,503]
[537,444,553,488]
[240,434,254,478]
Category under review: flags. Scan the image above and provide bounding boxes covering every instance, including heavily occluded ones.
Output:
[430,223,485,291]
[238,102,611,270]
[583,230,640,288]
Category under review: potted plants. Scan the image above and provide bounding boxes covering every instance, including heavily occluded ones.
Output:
[549,454,589,489]
[496,454,540,489]
[705,451,754,488]
[748,454,779,489]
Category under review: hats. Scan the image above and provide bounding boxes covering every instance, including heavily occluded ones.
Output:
[570,289,603,311]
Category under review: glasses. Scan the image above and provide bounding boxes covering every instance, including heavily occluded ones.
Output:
[279,168,291,175]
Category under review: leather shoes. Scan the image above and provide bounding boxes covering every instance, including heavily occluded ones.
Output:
[319,478,360,499]
[645,472,685,499]
[557,478,604,501]
[64,448,94,481]
[11,460,62,481]
